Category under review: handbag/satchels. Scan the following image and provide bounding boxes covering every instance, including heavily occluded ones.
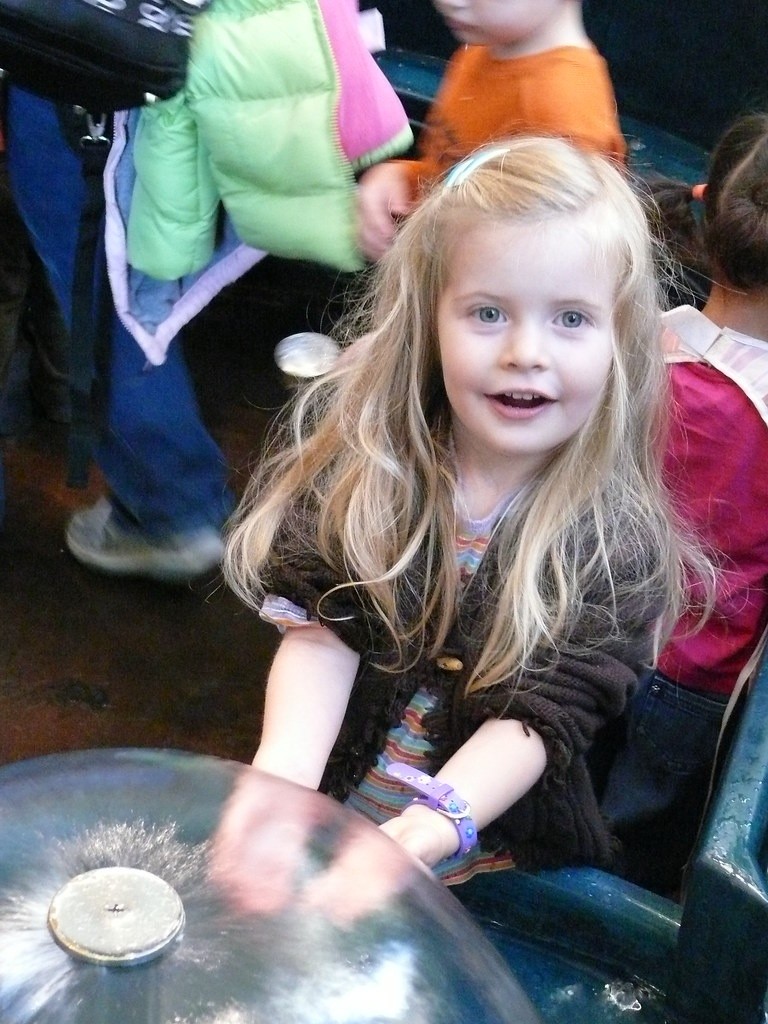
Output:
[0,0,201,114]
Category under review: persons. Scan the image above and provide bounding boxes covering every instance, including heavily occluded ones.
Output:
[1,1,293,472]
[586,110,768,899]
[205,132,763,1024]
[0,86,241,581]
[355,1,626,264]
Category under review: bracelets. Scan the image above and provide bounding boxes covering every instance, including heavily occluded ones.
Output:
[387,762,477,861]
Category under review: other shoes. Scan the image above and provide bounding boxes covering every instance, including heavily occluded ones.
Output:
[64,497,222,575]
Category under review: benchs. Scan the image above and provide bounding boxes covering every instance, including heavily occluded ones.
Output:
[359,0,768,310]
